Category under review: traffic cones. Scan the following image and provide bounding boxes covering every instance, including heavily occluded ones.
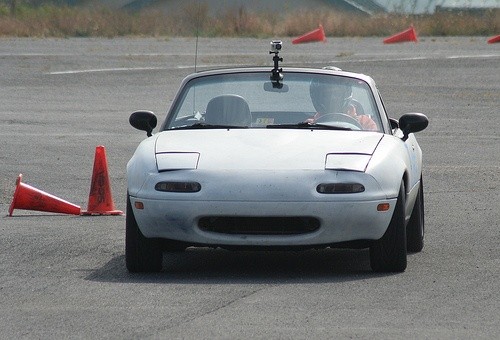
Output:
[487,34,500,44]
[290,22,326,44]
[80,145,124,216]
[8,174,82,217]
[384,25,419,44]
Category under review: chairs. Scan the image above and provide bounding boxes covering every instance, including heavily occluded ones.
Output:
[205,94,251,126]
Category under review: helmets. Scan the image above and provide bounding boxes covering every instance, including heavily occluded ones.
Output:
[309,65,352,115]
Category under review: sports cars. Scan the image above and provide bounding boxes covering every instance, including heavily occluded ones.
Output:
[124,66,431,274]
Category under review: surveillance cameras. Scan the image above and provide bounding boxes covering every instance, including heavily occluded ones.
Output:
[270,40,282,50]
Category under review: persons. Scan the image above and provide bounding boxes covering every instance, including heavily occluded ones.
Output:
[303,66,378,131]
